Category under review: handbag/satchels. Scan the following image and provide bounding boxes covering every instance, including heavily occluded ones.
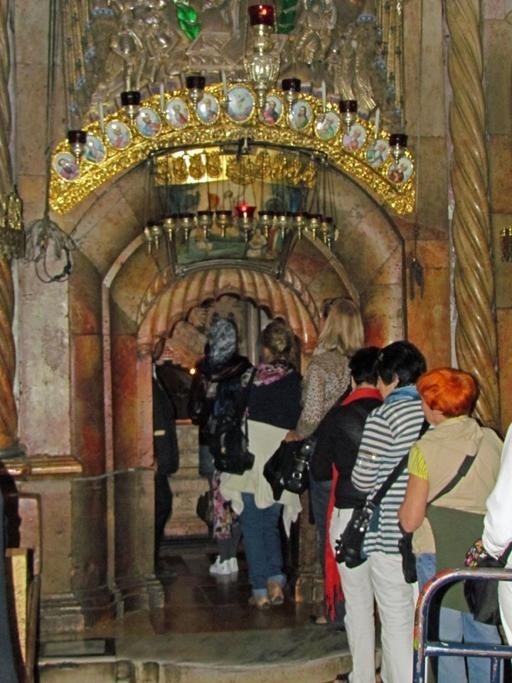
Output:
[278,439,316,494]
[398,531,417,583]
[157,360,194,419]
[463,537,512,624]
[333,504,374,568]
[209,421,254,474]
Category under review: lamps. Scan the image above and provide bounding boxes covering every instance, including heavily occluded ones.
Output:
[246,23,281,110]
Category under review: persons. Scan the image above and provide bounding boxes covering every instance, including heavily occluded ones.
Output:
[310,343,382,683]
[352,337,437,683]
[146,296,364,628]
[470,417,511,681]
[397,366,508,682]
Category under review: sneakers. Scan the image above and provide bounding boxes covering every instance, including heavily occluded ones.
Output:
[156,560,177,578]
[326,673,349,682]
[209,554,239,575]
[248,580,283,610]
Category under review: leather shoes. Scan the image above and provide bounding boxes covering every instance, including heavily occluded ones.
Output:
[309,614,330,625]
